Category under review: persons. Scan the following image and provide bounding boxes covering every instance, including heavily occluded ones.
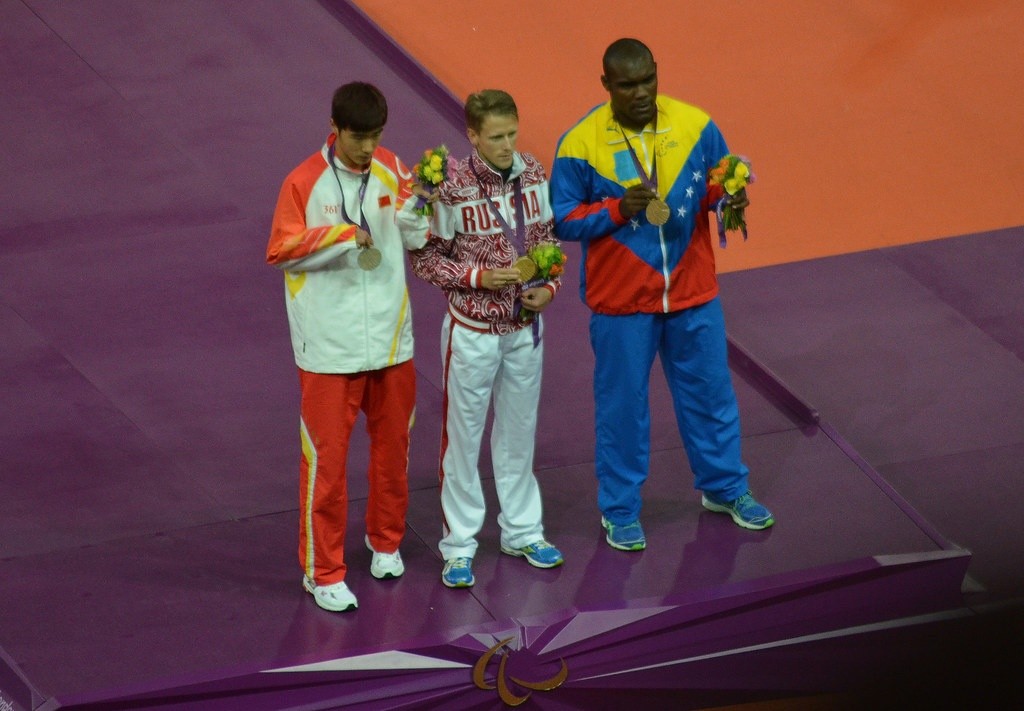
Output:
[549,38,774,550]
[265,82,442,612]
[408,89,565,589]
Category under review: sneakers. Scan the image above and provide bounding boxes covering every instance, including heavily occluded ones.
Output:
[501,540,563,568]
[302,576,358,612]
[702,490,776,529]
[365,534,405,579]
[602,516,646,550]
[442,557,475,587]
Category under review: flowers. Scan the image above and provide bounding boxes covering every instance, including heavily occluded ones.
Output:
[531,241,568,279]
[410,144,452,217]
[711,151,756,246]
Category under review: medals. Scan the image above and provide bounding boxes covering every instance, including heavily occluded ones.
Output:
[358,247,383,271]
[511,257,535,284]
[646,197,670,226]
